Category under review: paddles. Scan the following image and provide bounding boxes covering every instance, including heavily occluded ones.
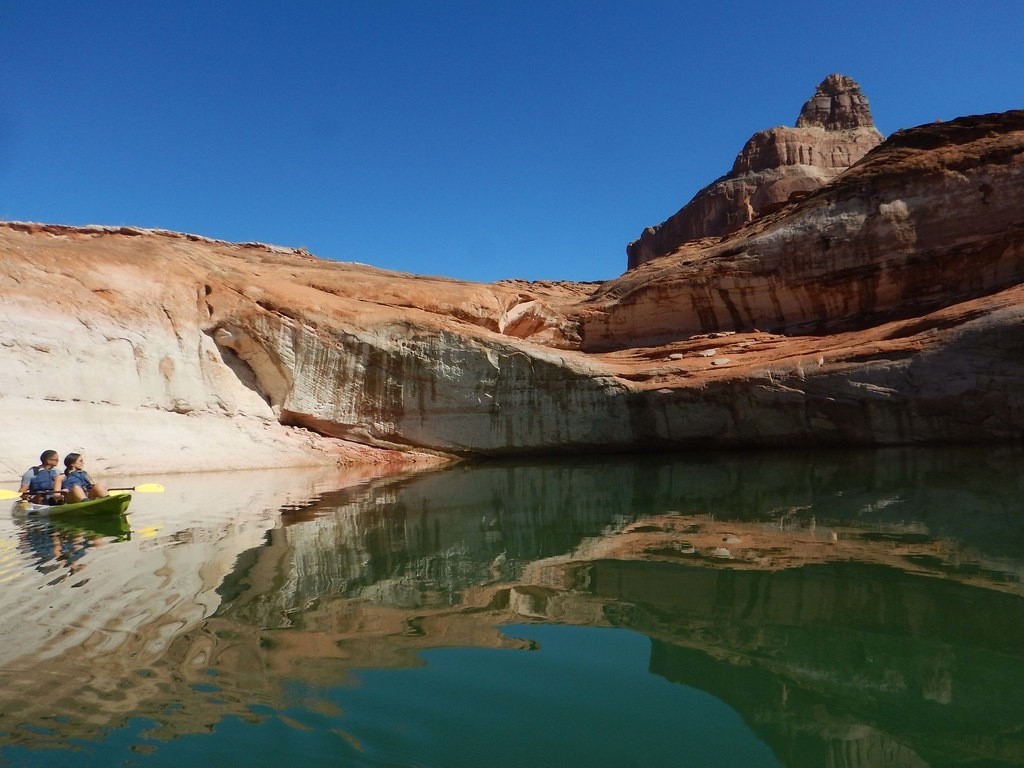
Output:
[0,482,164,502]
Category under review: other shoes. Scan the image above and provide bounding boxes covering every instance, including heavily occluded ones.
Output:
[81,497,100,502]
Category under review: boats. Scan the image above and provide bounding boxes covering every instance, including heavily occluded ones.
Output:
[13,488,132,519]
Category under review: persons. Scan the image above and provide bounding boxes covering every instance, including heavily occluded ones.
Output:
[19,450,59,504]
[53,453,110,504]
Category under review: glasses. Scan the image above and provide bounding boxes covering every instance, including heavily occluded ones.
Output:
[49,458,58,462]
